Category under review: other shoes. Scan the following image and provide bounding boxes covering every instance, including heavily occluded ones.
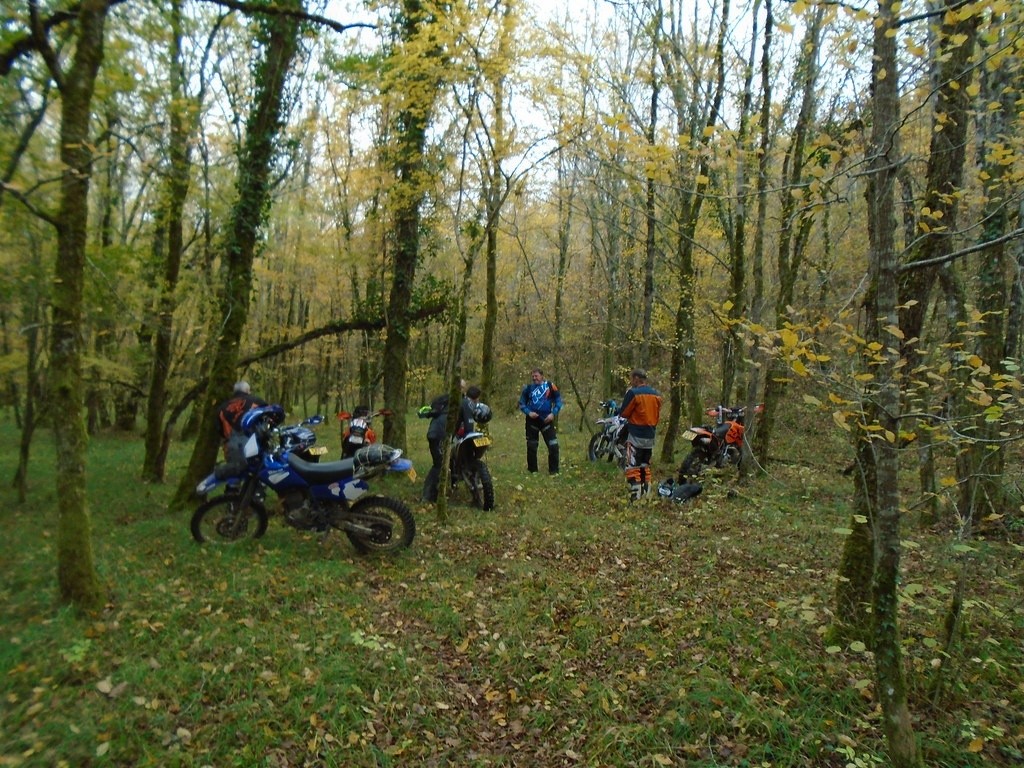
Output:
[548,468,559,475]
[422,496,450,505]
[528,467,538,474]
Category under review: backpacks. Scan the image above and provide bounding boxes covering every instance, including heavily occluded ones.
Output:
[430,394,448,418]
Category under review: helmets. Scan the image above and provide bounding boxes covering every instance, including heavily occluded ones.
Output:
[280,425,316,449]
[241,404,285,438]
[473,403,492,425]
[349,419,369,437]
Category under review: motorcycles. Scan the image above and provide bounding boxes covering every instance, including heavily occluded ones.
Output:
[419,403,494,511]
[588,401,628,470]
[338,405,393,461]
[678,403,763,488]
[271,416,324,466]
[189,414,419,557]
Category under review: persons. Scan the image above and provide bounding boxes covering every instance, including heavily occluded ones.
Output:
[217,381,276,518]
[519,368,562,478]
[619,368,663,508]
[422,387,481,506]
